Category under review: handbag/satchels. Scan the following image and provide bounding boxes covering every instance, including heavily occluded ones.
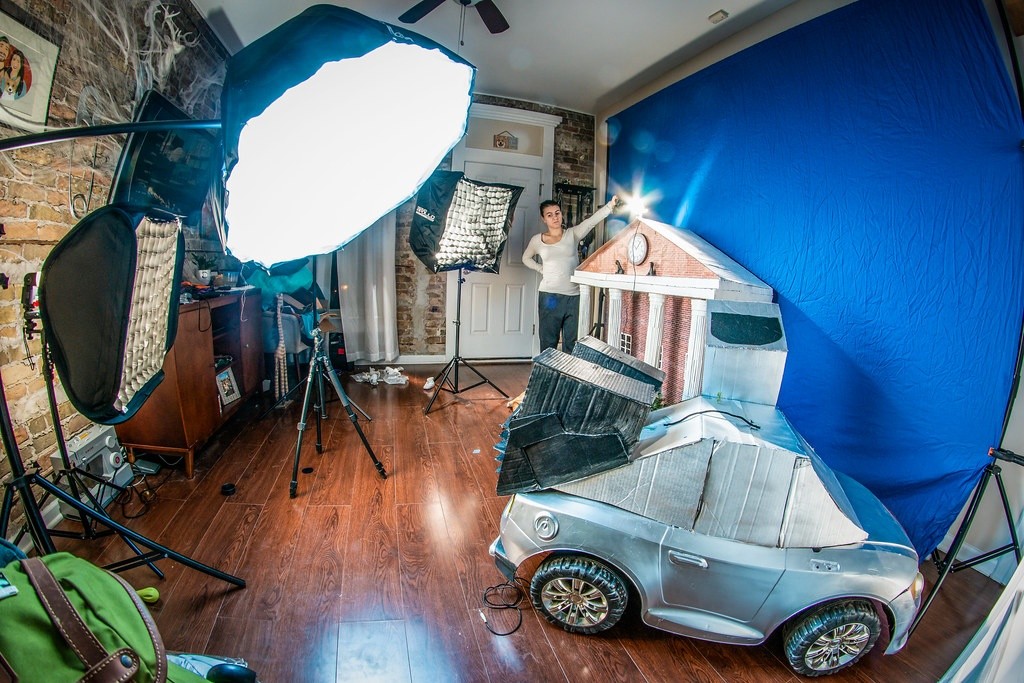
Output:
[0,551,211,683]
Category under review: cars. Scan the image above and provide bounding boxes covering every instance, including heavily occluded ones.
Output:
[489,393,925,677]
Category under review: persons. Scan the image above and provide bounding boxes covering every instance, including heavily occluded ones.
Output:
[522,193,621,353]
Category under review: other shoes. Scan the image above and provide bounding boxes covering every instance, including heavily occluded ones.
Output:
[423,376,436,390]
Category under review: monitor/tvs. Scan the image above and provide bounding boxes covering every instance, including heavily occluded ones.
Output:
[106,90,221,226]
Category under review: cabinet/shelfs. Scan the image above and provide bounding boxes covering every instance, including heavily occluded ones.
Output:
[113,286,263,479]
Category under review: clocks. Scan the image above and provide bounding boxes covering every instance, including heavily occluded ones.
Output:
[628,233,648,265]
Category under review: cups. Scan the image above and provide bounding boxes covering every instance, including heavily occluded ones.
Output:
[220,271,239,287]
